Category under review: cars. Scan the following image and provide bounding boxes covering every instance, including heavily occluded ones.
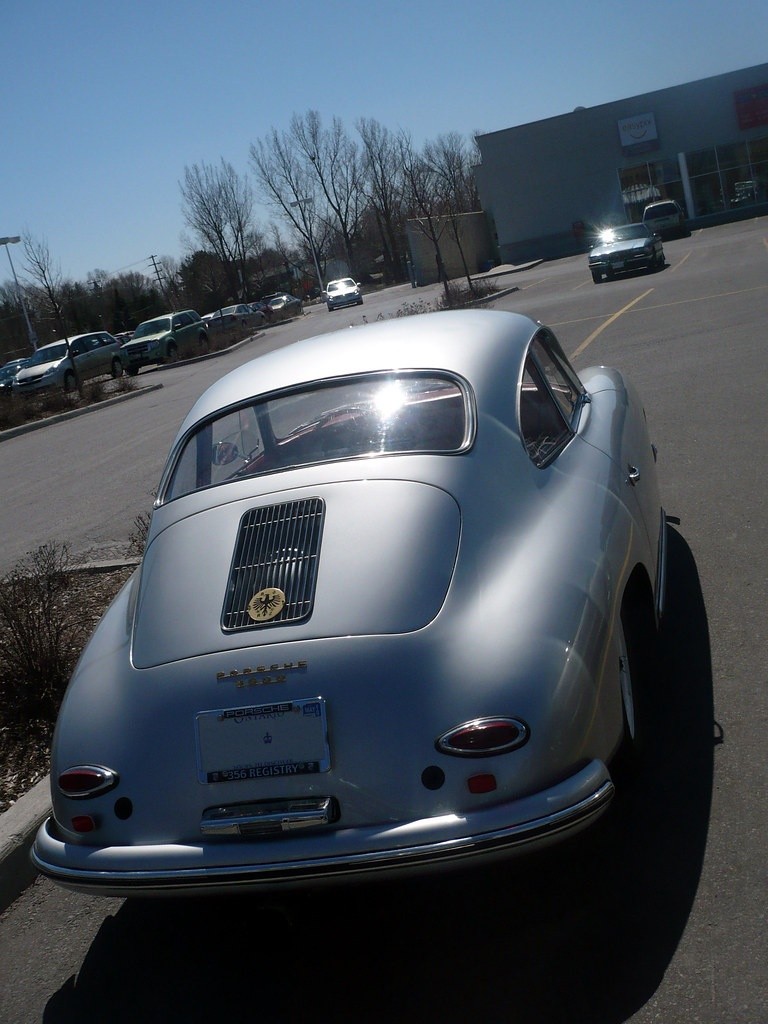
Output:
[587,221,666,285]
[0,329,137,406]
[323,278,363,312]
[29,310,678,903]
[200,292,303,350]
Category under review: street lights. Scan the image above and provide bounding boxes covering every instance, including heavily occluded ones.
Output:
[290,197,324,292]
[0,235,39,351]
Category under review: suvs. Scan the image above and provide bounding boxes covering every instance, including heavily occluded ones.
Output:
[119,309,210,376]
[642,198,689,239]
